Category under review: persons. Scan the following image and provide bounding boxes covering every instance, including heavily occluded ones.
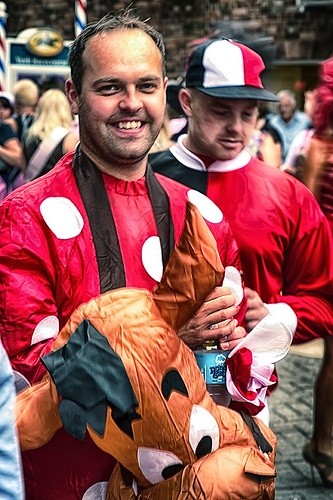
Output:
[147,38,333,428]
[301,55,333,500]
[0,80,333,203]
[1,14,271,500]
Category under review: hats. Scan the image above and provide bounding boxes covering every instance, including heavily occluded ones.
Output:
[0,91,16,115]
[185,37,280,102]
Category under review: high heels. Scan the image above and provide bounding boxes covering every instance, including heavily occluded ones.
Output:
[302,443,333,490]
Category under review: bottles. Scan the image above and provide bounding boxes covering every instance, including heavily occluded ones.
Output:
[192,340,230,407]
